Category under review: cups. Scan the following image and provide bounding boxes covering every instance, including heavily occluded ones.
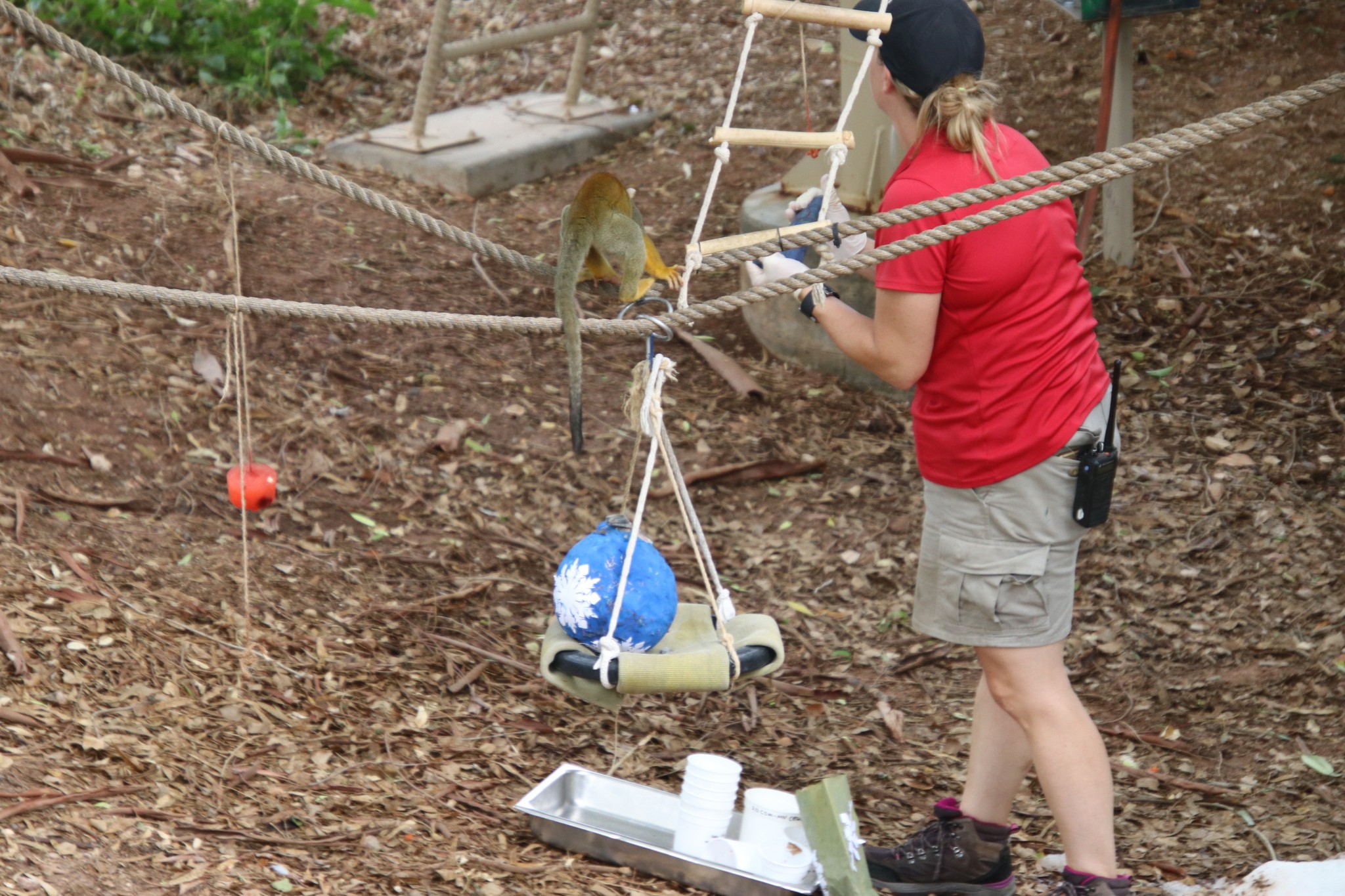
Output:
[739,786,801,873]
[672,754,744,862]
[769,842,810,885]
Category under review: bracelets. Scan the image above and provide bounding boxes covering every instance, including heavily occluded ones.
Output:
[798,283,840,323]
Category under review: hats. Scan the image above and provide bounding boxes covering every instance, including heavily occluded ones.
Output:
[849,0,985,99]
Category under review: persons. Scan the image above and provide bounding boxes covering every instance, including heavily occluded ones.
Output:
[744,1,1131,895]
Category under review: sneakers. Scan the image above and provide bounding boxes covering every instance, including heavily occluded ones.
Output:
[865,799,1019,895]
[1051,866,1134,896]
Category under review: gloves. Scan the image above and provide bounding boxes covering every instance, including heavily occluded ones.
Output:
[746,252,810,305]
[785,175,867,263]
